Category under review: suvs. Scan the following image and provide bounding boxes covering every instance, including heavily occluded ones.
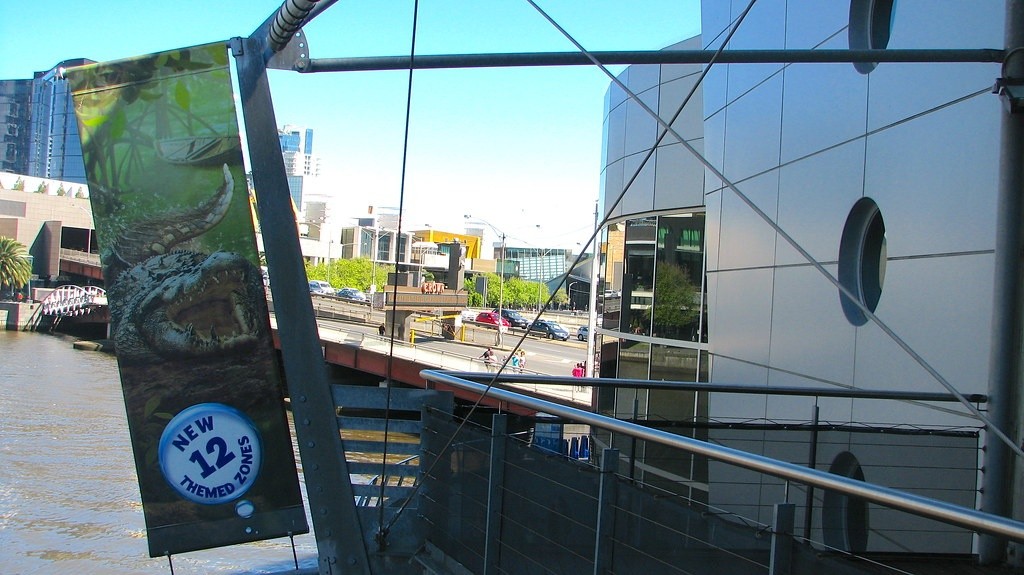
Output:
[492,308,530,329]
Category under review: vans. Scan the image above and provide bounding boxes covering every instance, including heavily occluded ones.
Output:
[309,280,334,298]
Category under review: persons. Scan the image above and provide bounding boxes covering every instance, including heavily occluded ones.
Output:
[479,347,492,372]
[378,323,385,341]
[519,351,526,374]
[487,350,497,373]
[572,361,587,392]
[511,353,520,373]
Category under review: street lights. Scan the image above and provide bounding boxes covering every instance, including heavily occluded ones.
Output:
[402,230,450,295]
[71,203,92,253]
[568,282,578,310]
[464,215,506,347]
[343,218,401,320]
[327,243,368,293]
[34,67,64,178]
[538,242,580,313]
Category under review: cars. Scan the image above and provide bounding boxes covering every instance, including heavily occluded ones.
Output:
[335,288,366,305]
[559,324,570,339]
[577,326,598,341]
[475,312,511,330]
[461,307,476,322]
[527,321,568,341]
[263,275,270,287]
